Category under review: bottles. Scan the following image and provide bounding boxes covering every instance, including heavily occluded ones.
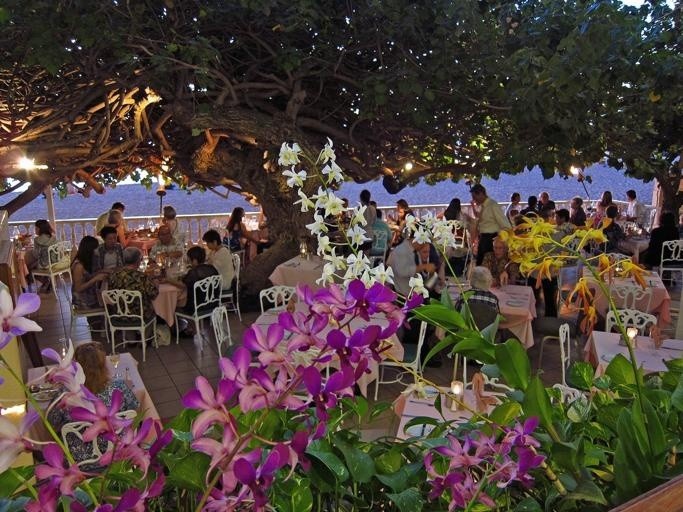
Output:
[146,216,152,226]
[13,226,20,247]
[123,367,132,386]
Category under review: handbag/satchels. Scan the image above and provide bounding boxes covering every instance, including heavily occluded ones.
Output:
[151,323,171,348]
[52,256,69,273]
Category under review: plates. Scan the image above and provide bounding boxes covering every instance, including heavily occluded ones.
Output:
[507,300,524,307]
[31,389,56,401]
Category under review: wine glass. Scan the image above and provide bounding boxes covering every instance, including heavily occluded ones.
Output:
[142,256,149,265]
[110,351,120,377]
[150,226,156,236]
[139,261,145,271]
[139,224,145,230]
[128,227,133,237]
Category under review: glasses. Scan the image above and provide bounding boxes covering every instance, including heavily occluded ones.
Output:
[492,245,503,251]
[157,232,169,236]
[540,196,548,200]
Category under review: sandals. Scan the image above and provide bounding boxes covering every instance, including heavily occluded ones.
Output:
[175,330,193,338]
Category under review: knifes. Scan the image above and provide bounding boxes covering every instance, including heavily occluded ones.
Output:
[402,415,438,420]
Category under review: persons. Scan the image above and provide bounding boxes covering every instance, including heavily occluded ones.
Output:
[96,226,125,271]
[222,206,269,265]
[256,208,274,254]
[29,219,59,292]
[641,210,680,287]
[318,183,642,369]
[69,234,109,311]
[149,224,185,261]
[178,246,220,339]
[94,201,129,234]
[97,209,133,248]
[201,228,235,292]
[44,339,144,480]
[161,204,180,245]
[108,246,160,348]
